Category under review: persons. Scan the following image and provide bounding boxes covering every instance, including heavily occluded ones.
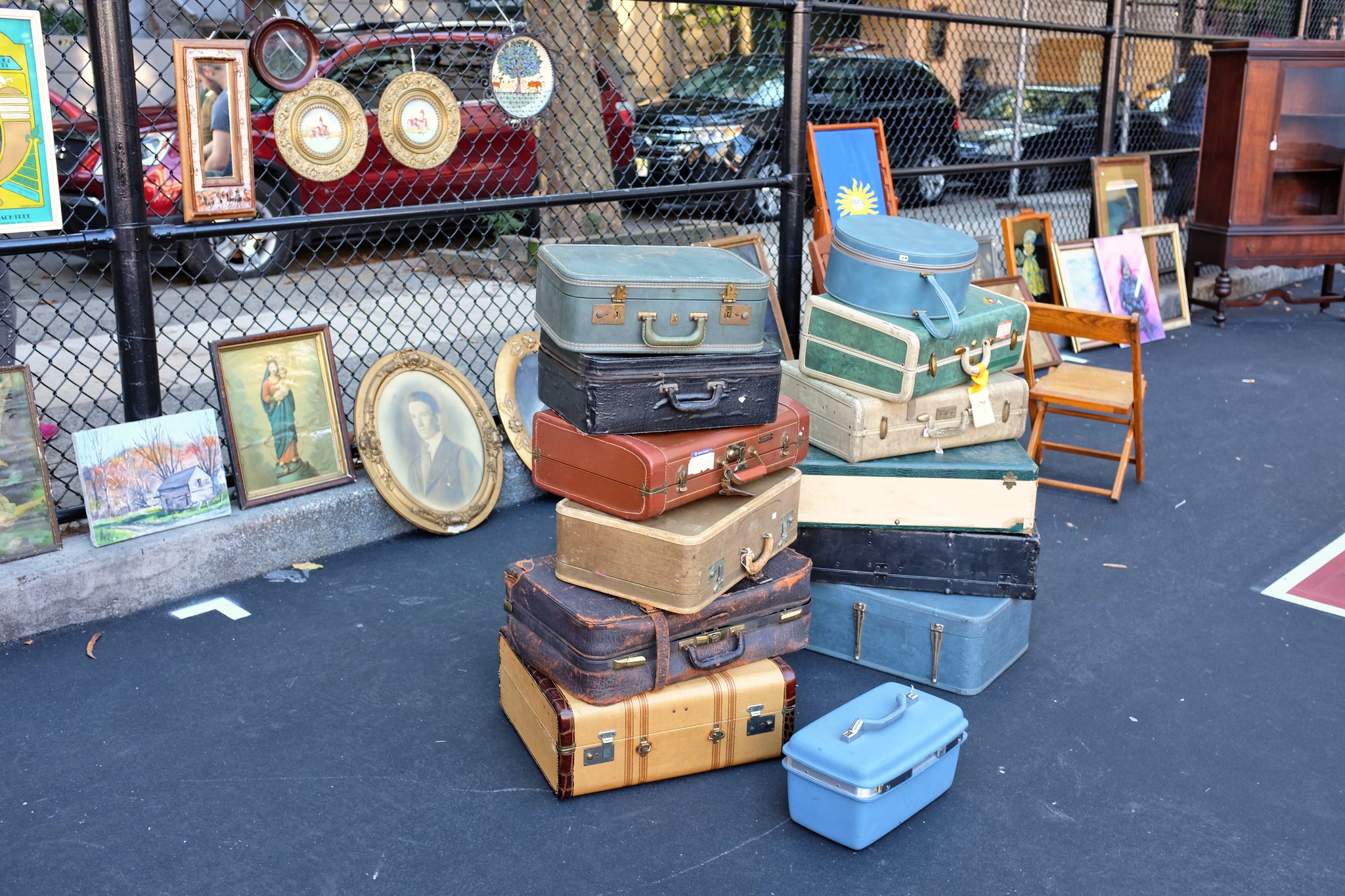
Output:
[1160,55,1208,228]
[1207,8,1345,41]
[195,57,232,178]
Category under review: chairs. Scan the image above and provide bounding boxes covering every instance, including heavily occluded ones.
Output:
[1023,296,1156,508]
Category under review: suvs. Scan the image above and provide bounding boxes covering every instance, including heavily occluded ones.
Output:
[632,52,961,222]
[43,17,637,285]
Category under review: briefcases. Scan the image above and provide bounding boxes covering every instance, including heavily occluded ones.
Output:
[531,395,810,519]
[781,681,969,851]
[827,213,980,341]
[534,244,771,356]
[776,359,1030,464]
[804,580,1032,695]
[535,328,784,436]
[498,629,799,800]
[793,444,1038,538]
[505,548,815,708]
[791,523,1041,602]
[554,466,801,614]
[798,286,1030,403]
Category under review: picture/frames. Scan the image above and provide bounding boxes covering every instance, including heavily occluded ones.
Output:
[970,274,1064,374]
[1087,153,1164,311]
[492,329,540,466]
[973,234,1003,281]
[999,207,1061,309]
[806,115,900,234]
[213,321,355,514]
[355,347,506,535]
[696,229,795,359]
[0,8,64,235]
[1120,221,1193,333]
[171,36,260,219]
[0,362,65,565]
[1049,233,1121,354]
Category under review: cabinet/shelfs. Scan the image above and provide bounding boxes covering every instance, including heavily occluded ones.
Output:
[1181,34,1345,331]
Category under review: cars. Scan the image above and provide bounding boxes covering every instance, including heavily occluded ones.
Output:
[956,66,1193,194]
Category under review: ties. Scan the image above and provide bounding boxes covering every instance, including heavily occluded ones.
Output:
[419,442,432,494]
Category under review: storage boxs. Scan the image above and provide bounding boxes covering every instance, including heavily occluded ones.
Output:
[494,625,799,797]
[794,523,1044,602]
[792,444,1041,536]
[804,581,1032,698]
